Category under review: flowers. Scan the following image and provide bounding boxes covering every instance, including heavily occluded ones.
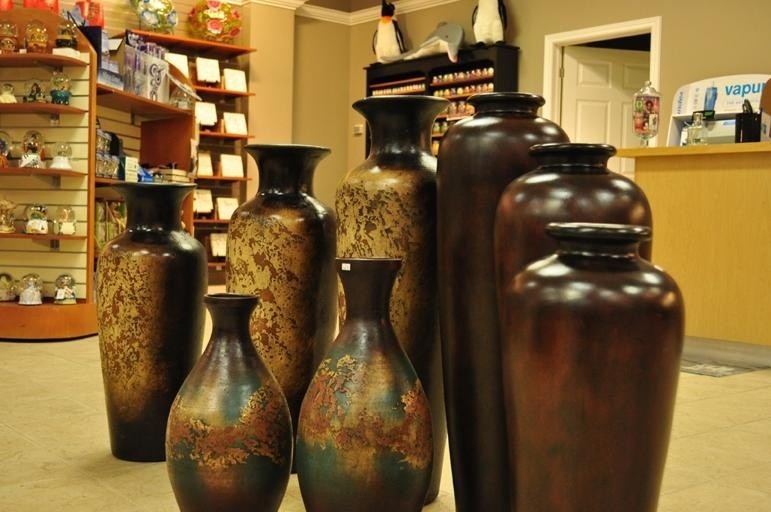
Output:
[128,0,179,34]
[186,0,241,44]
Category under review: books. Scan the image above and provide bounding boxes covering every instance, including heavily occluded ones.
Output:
[163,173,192,184]
[159,168,188,177]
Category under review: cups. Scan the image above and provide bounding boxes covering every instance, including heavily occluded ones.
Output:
[735,113,761,143]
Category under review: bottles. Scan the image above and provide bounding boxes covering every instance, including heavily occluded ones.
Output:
[295,248,437,512]
[686,111,710,145]
[221,138,338,361]
[442,87,695,512]
[163,293,294,512]
[337,92,439,396]
[96,168,209,467]
[633,80,660,148]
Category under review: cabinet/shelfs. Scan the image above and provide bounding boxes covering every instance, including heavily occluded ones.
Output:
[92,59,196,333]
[362,44,520,159]
[0,8,99,340]
[108,30,258,286]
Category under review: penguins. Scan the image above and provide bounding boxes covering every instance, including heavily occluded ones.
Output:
[372,0,405,62]
[471,0,507,48]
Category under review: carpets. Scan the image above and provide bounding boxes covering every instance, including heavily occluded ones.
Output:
[681,335,771,377]
